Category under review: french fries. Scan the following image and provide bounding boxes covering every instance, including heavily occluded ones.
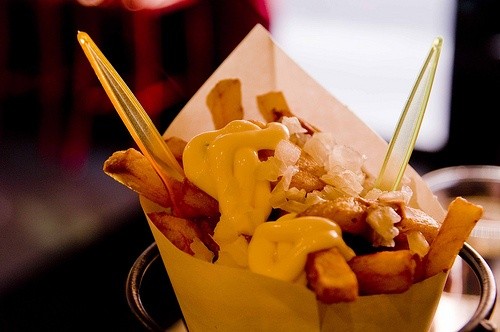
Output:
[102,23,485,331]
